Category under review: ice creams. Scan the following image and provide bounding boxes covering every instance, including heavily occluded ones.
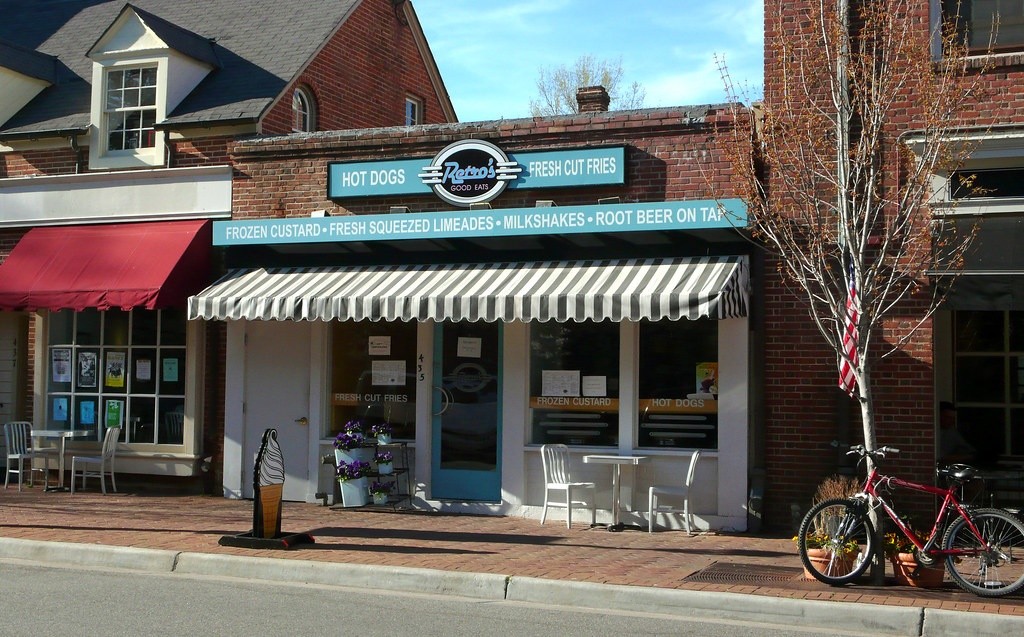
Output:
[252,429,284,539]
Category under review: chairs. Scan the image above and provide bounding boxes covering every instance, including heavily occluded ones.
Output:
[540,444,596,530]
[71,427,120,495]
[3,421,48,492]
[648,451,699,535]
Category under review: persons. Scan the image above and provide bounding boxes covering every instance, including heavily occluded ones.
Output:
[940,400,974,482]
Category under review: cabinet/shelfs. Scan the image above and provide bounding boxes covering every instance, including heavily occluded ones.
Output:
[338,441,412,509]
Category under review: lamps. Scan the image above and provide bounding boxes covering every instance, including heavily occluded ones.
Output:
[469,203,492,209]
[390,206,411,213]
[311,210,330,217]
[536,200,557,207]
[598,196,620,203]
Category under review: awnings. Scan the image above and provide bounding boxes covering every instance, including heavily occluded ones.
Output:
[188,253,753,322]
[0,218,212,312]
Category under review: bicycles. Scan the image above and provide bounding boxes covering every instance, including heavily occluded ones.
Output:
[798,445,1024,597]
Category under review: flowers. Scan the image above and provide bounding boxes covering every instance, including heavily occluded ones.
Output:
[370,479,396,495]
[791,531,862,561]
[882,530,965,567]
[335,460,371,483]
[371,451,394,464]
[372,424,394,437]
[332,422,367,452]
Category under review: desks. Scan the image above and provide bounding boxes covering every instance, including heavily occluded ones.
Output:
[30,429,95,494]
[583,454,650,532]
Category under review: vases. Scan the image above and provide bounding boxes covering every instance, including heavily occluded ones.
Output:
[800,548,853,579]
[891,553,945,588]
[334,436,394,508]
